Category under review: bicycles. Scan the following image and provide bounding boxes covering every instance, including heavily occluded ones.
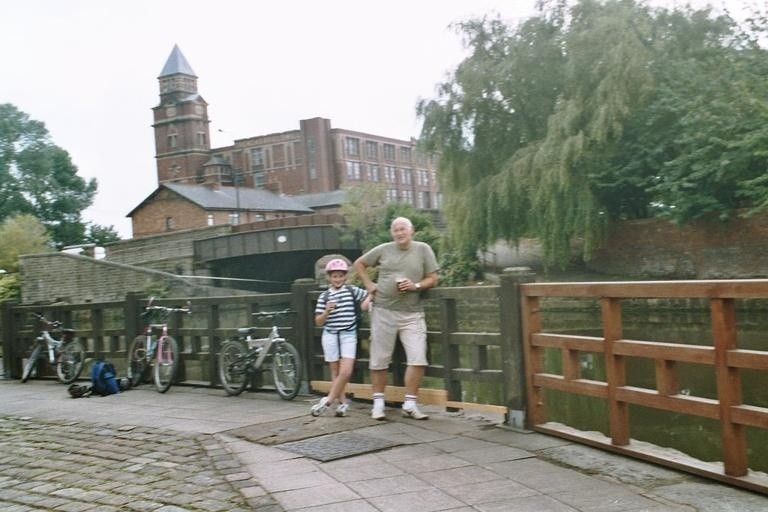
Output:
[20,313,86,385]
[218,308,302,401]
[127,297,193,393]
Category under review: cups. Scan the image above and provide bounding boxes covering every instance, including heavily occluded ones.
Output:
[396,278,409,296]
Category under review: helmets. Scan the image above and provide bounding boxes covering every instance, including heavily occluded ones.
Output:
[325,258,349,274]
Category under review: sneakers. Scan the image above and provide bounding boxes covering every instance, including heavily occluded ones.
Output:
[335,398,348,416]
[371,405,386,419]
[401,406,429,420]
[311,396,331,416]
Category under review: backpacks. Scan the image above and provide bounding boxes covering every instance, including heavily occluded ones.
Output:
[91,360,118,397]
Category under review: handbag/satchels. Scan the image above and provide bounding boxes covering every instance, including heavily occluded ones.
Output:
[68,384,92,397]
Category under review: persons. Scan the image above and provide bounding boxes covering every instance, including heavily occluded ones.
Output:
[311,259,373,418]
[353,216,440,421]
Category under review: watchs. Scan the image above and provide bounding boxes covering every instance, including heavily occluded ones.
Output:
[414,282,420,292]
